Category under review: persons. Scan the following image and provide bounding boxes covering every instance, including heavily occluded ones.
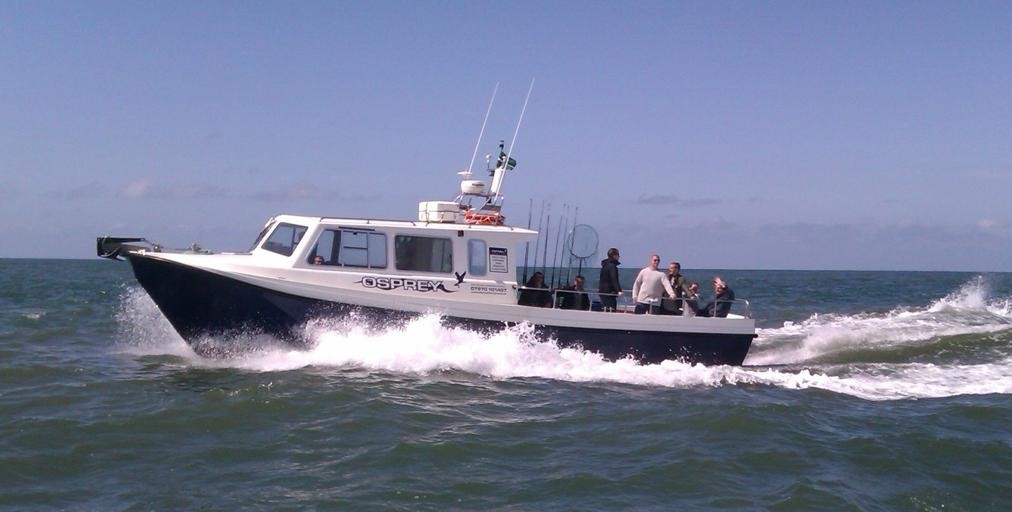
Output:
[661,262,696,316]
[313,256,325,265]
[598,248,624,312]
[632,255,677,314]
[553,275,590,310]
[682,282,705,316]
[705,276,735,317]
[518,272,553,308]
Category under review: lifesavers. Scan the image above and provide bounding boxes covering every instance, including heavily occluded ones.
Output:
[465,215,504,224]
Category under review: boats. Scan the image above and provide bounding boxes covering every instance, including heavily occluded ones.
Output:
[94,75,763,373]
